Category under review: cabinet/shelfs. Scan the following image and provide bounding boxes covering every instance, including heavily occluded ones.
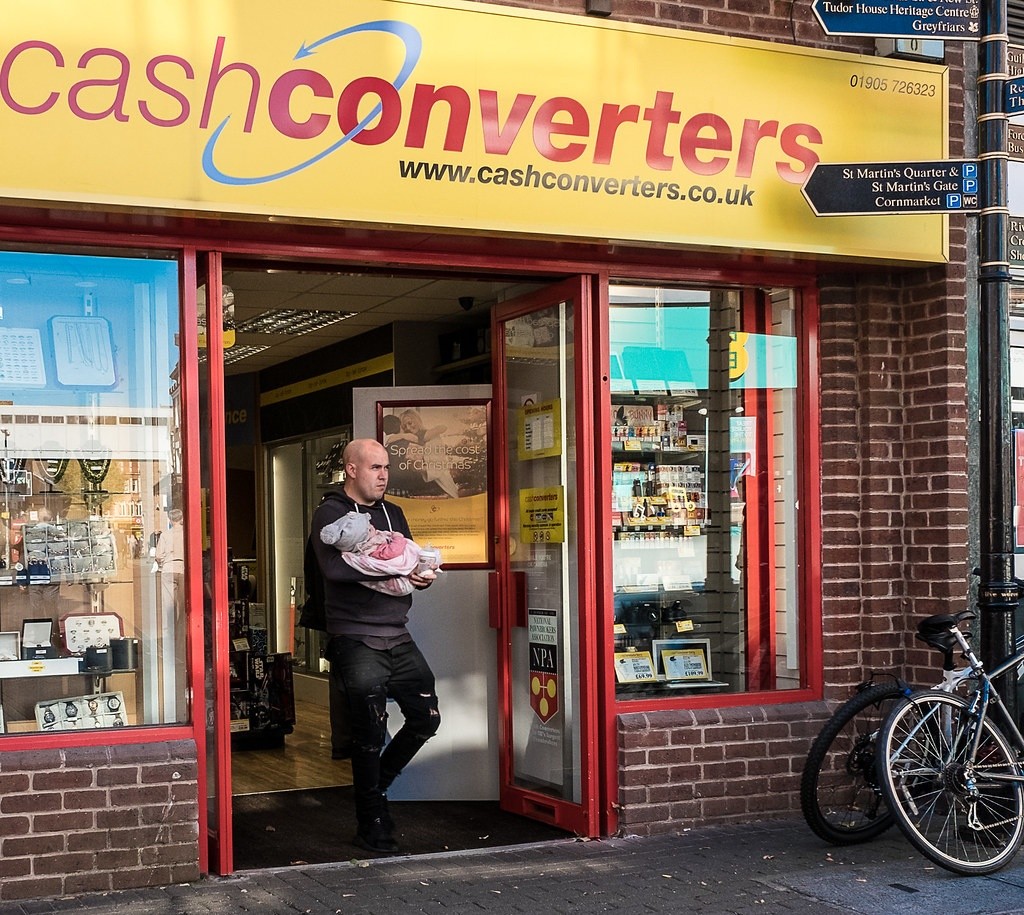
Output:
[611,393,729,694]
[0,491,140,679]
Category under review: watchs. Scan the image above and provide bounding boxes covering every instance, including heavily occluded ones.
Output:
[43,695,124,731]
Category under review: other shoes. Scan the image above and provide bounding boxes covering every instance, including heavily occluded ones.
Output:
[352,816,399,853]
[378,788,395,830]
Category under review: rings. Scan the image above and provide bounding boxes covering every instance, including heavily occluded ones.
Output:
[69,619,114,649]
[0,334,39,380]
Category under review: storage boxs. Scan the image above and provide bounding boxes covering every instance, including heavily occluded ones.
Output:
[21,618,58,660]
[0,657,79,679]
[0,631,21,661]
[249,652,296,730]
[611,403,688,451]
[21,519,117,583]
[612,463,705,525]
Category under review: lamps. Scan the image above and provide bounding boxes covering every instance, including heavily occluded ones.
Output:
[197,284,235,348]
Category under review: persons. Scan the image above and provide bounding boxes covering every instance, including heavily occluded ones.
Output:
[305,438,441,852]
[384,409,460,501]
[153,508,186,636]
[321,511,442,595]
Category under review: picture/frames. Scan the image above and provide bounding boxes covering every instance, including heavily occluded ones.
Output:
[652,638,712,683]
[375,398,497,571]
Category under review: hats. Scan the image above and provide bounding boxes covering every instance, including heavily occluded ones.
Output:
[320,511,371,552]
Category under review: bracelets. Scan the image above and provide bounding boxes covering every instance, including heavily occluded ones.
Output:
[25,528,117,573]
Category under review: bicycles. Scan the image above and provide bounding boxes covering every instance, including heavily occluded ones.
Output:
[801,566,1024,876]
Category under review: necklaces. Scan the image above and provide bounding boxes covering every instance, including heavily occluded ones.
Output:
[0,446,112,484]
[64,321,110,373]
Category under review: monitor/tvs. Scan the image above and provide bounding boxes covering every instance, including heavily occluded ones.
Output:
[652,639,711,681]
